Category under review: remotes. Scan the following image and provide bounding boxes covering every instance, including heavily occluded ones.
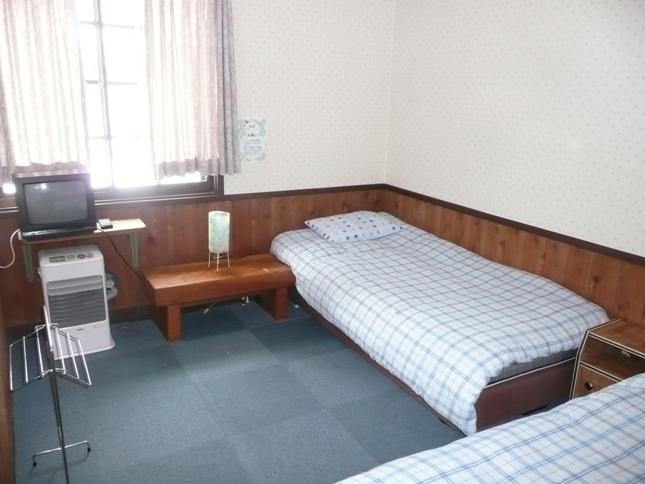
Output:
[98,218,113,229]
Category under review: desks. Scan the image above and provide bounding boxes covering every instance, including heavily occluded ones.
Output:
[140,254,294,341]
[570,318,645,396]
[16,219,148,283]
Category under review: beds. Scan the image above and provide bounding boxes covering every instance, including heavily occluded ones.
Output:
[334,378,645,483]
[270,213,609,431]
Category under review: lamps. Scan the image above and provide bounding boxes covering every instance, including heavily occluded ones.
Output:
[207,209,230,271]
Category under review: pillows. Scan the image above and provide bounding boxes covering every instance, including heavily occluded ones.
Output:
[305,209,401,245]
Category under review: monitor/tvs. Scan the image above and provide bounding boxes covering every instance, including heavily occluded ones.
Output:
[14,168,97,241]
